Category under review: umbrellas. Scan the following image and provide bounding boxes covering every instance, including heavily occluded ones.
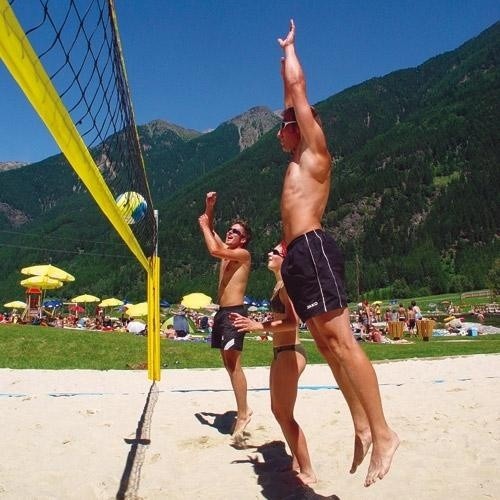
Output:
[3,263,213,335]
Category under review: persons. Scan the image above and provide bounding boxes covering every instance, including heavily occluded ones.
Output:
[276,17,402,488]
[227,237,318,491]
[3,306,112,331]
[348,300,484,342]
[247,312,273,323]
[197,190,253,438]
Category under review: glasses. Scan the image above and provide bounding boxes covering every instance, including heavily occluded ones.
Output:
[282,121,296,128]
[270,248,282,257]
[228,229,241,236]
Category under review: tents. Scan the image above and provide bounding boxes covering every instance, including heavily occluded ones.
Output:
[159,313,195,334]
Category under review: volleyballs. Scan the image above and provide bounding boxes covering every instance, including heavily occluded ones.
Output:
[115,191,147,224]
